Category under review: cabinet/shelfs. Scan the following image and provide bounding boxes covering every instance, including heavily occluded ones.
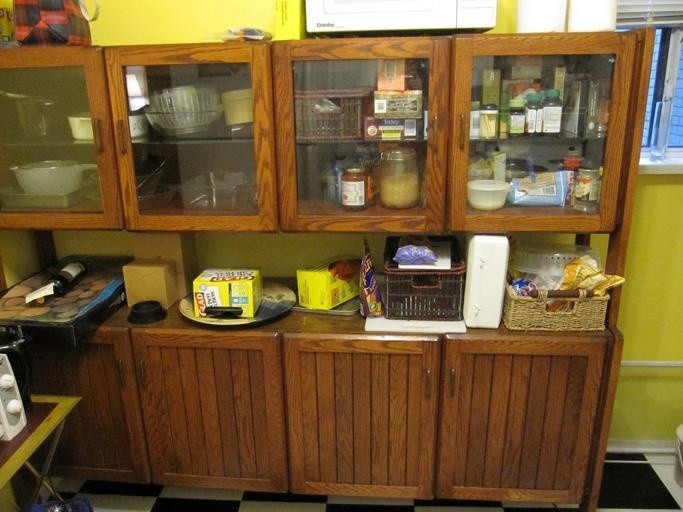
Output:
[270,26,654,234]
[282,307,624,511]
[0,43,279,232]
[35,315,286,491]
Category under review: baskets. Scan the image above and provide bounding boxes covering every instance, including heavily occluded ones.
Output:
[502,275,610,332]
[380,236,466,321]
[293,87,375,139]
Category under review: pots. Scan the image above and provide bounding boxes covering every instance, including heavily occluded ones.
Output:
[13,160,97,197]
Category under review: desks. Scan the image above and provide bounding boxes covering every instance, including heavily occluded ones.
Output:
[1,393,84,510]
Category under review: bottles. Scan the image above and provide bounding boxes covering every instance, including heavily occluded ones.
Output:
[478,103,499,139]
[377,145,422,209]
[572,165,600,214]
[35,260,88,304]
[228,28,272,41]
[507,88,563,138]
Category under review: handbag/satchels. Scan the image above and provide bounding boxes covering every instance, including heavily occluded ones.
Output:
[12,0,100,46]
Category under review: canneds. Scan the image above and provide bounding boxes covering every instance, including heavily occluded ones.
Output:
[506,159,528,184]
[340,168,368,213]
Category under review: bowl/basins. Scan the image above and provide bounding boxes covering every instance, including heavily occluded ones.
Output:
[467,180,511,211]
[134,163,163,195]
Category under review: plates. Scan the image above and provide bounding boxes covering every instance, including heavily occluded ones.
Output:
[179,281,297,327]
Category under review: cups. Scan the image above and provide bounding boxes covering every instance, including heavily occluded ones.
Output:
[650,101,672,160]
[15,97,58,142]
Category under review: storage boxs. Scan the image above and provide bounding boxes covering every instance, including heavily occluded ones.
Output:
[293,255,359,309]
[127,228,197,297]
[121,257,179,309]
[191,268,263,317]
